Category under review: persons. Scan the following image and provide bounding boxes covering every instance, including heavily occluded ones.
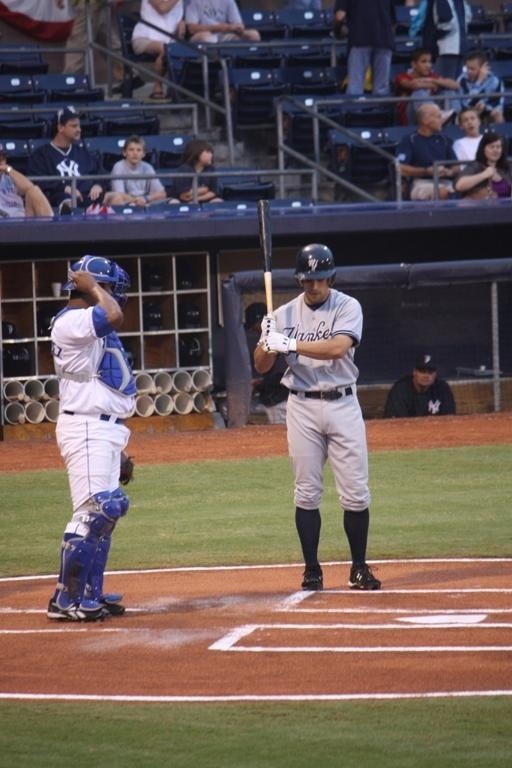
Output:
[252,243,383,589]
[48,256,139,623]
[168,137,224,204]
[110,133,180,207]
[395,102,512,201]
[1,150,54,218]
[452,50,506,126]
[21,107,111,216]
[282,0,473,113]
[131,0,260,99]
[55,0,124,85]
[393,46,460,126]
[245,302,289,424]
[385,354,457,418]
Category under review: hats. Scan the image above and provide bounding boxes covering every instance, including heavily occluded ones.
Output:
[414,353,436,372]
[51,104,87,131]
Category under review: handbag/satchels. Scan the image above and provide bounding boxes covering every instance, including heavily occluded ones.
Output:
[3,343,32,376]
[177,301,202,330]
[141,262,168,292]
[175,264,198,290]
[1,321,20,338]
[179,334,205,366]
[143,300,164,331]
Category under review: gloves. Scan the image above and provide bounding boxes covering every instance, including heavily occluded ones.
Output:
[259,331,292,357]
[259,315,277,338]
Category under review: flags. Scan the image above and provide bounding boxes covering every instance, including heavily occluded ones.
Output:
[83,203,116,215]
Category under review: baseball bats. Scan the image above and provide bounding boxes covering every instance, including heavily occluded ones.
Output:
[257,199,273,315]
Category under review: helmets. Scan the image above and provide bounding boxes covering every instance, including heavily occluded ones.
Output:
[59,254,132,310]
[293,242,337,281]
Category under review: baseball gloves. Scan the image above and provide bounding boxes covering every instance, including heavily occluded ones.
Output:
[121,452,133,486]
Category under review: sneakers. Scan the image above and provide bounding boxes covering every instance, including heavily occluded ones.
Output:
[44,596,109,623]
[301,563,324,591]
[347,567,382,590]
[85,596,127,618]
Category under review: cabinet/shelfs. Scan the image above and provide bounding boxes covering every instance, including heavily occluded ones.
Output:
[0,249,219,425]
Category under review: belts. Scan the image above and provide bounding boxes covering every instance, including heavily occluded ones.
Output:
[290,386,354,401]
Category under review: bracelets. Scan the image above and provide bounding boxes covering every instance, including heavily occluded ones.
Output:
[143,195,150,203]
[6,166,12,174]
[288,338,296,351]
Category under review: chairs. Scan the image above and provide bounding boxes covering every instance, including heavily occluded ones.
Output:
[1,1,512,230]
